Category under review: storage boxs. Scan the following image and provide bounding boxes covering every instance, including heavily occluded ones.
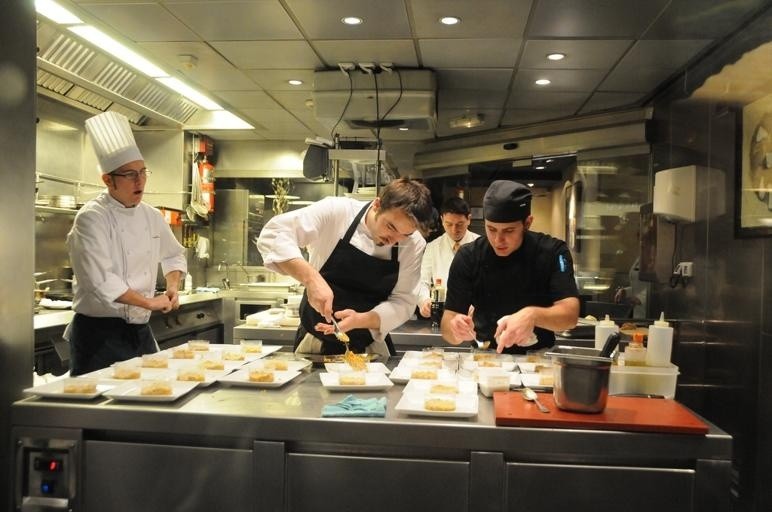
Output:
[608,361,680,400]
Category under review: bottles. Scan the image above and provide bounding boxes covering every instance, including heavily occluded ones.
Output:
[431,278,446,326]
[625,333,647,366]
[185,271,193,294]
[594,314,620,364]
[648,312,675,365]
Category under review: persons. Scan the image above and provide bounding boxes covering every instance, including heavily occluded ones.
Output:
[613,258,647,319]
[62,111,187,376]
[416,204,437,240]
[415,196,481,318]
[256,175,426,354]
[440,180,581,354]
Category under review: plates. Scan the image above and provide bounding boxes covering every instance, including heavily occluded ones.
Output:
[302,353,383,365]
[40,303,73,310]
[389,351,555,419]
[24,339,309,404]
[320,372,394,391]
[343,187,377,200]
[39,194,78,208]
[324,361,392,375]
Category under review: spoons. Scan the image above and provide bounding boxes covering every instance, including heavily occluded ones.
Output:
[330,316,350,341]
[467,329,491,350]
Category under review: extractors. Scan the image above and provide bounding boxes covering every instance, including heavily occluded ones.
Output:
[37,5,203,130]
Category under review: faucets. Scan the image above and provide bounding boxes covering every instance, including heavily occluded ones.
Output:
[233,261,252,282]
[217,261,231,290]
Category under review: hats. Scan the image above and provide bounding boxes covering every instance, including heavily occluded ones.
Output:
[85,110,144,175]
[482,179,533,222]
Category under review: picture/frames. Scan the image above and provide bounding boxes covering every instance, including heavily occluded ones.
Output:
[732,80,772,239]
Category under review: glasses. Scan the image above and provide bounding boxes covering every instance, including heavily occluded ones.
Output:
[115,170,152,181]
